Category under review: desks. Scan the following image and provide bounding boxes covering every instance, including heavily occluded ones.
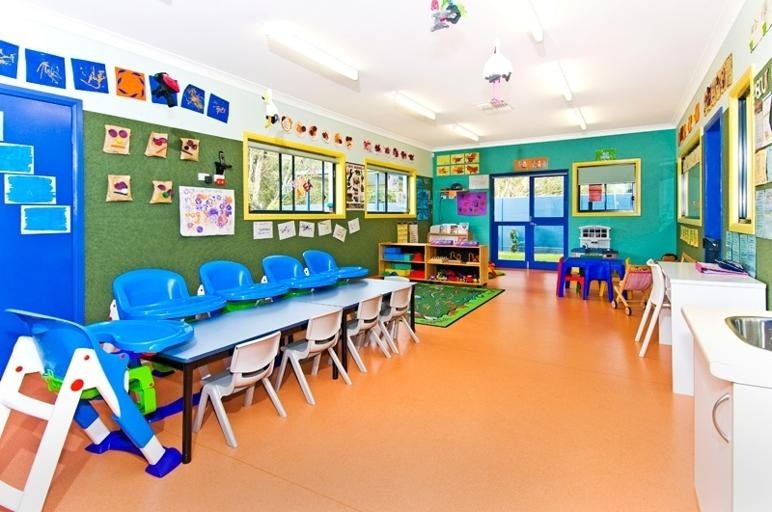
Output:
[658,261,766,394]
[120,277,421,465]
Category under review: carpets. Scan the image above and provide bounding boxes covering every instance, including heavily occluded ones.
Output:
[405,282,506,328]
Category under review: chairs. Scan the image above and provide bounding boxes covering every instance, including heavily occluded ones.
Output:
[346,293,391,373]
[1,300,178,512]
[113,266,188,320]
[303,249,337,273]
[379,285,419,354]
[192,331,287,449]
[635,259,671,358]
[583,259,613,301]
[198,261,255,291]
[261,254,304,281]
[556,256,584,298]
[274,306,351,407]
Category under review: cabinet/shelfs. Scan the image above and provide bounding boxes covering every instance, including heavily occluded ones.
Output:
[377,242,482,286]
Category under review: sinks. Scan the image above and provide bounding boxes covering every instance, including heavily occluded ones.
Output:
[725,315,772,352]
